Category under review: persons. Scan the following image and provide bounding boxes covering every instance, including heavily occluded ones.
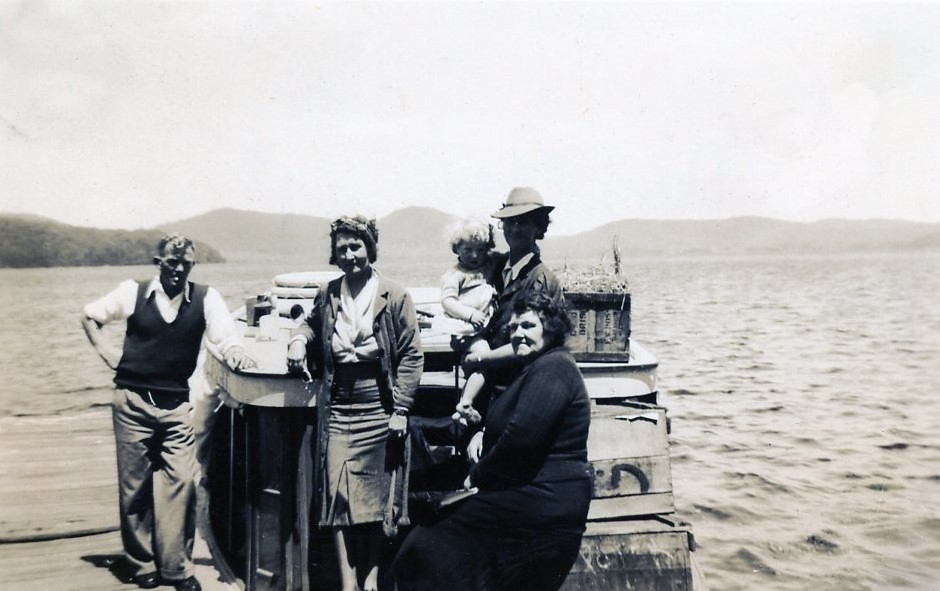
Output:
[442,186,566,440]
[285,215,424,591]
[376,293,592,591]
[81,233,257,591]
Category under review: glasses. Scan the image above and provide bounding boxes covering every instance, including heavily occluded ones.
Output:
[497,215,531,230]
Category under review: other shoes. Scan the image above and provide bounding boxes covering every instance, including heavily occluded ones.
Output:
[452,402,482,431]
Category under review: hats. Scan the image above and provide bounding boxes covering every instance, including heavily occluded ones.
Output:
[490,186,556,219]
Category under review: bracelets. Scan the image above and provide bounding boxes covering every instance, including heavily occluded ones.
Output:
[393,409,407,416]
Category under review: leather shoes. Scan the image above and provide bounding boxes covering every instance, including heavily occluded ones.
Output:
[174,576,202,591]
[136,572,160,589]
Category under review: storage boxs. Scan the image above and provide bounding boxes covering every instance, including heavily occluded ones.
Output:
[557,513,696,591]
[559,290,633,363]
[586,403,674,521]
